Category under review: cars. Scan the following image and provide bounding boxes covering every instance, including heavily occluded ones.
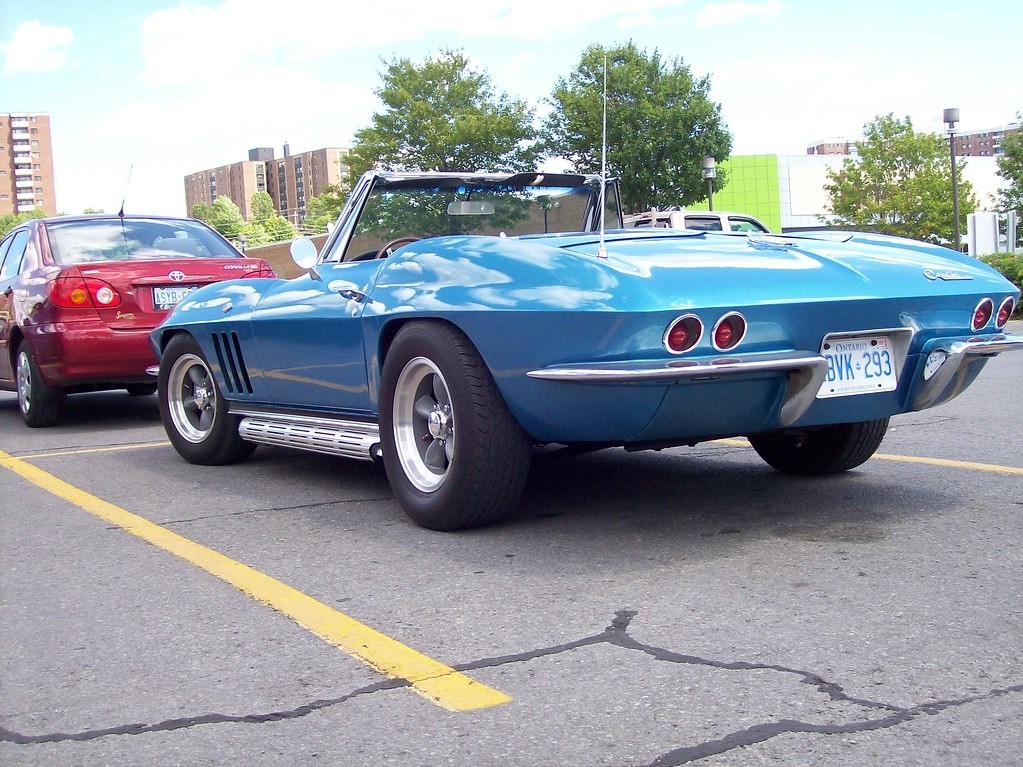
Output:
[1,213,280,429]
[146,172,1023,533]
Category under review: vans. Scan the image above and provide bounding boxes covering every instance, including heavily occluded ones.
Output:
[620,208,773,235]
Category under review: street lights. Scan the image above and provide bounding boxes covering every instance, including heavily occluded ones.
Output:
[943,108,962,252]
[701,158,716,211]
